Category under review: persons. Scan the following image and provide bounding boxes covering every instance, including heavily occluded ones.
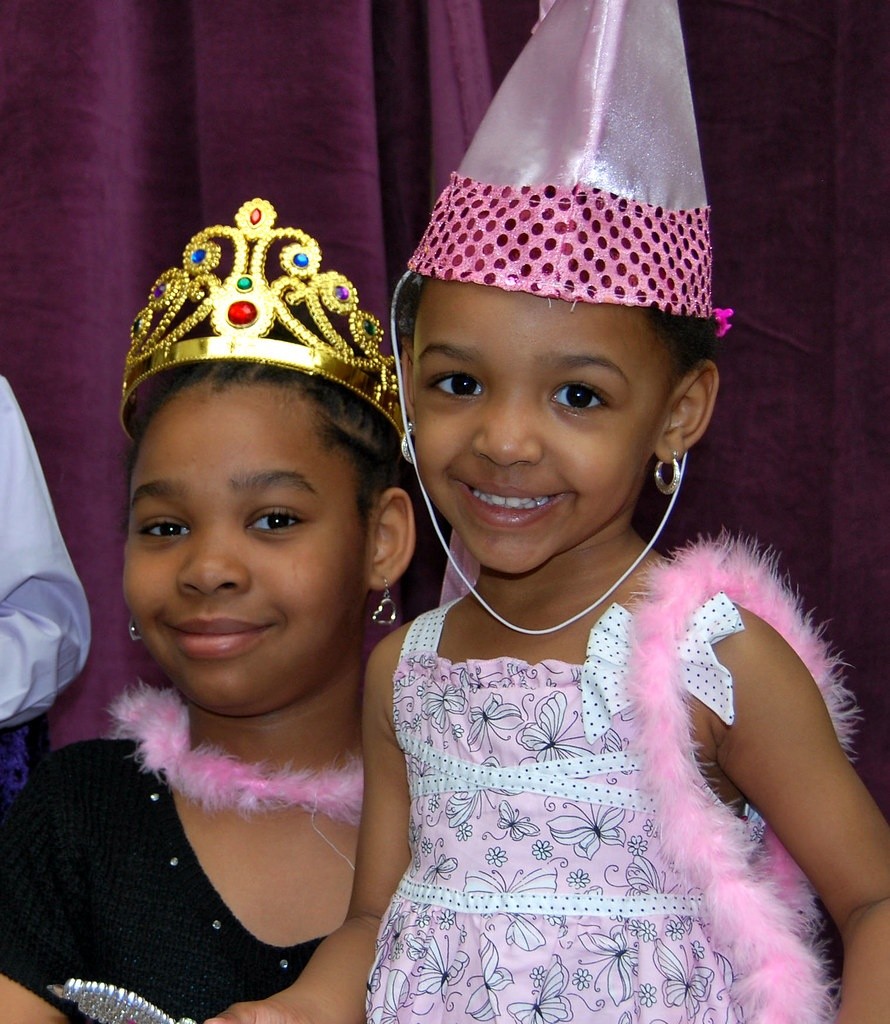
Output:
[1,377,92,805]
[1,313,426,1023]
[198,135,890,1023]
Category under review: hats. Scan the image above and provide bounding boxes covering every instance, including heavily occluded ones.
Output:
[408,0,713,320]
